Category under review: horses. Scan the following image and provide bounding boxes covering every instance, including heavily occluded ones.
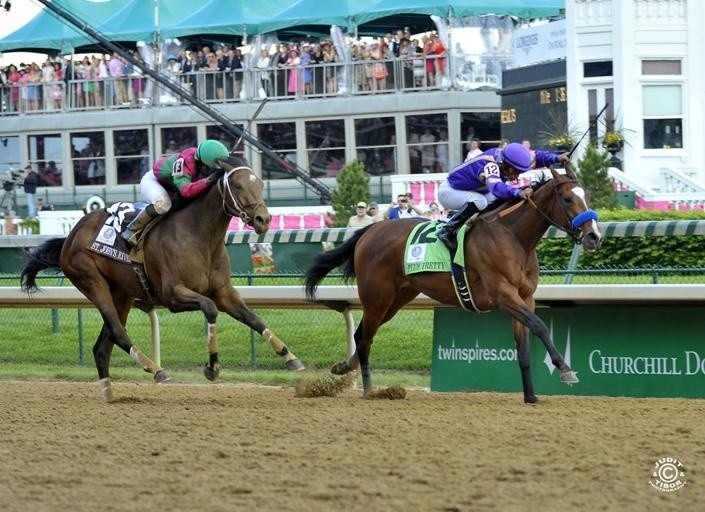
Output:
[19,156,307,404]
[303,159,605,404]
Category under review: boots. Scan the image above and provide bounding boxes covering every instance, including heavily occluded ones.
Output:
[121,203,159,247]
[434,201,479,251]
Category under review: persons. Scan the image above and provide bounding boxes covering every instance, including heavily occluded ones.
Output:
[434,142,573,249]
[34,199,53,210]
[120,139,229,246]
[0,29,499,113]
[39,124,534,186]
[344,193,453,240]
[22,165,39,215]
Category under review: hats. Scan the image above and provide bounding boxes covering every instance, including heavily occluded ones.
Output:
[357,202,367,208]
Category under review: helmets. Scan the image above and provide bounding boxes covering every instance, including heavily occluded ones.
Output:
[196,139,228,172]
[500,142,532,172]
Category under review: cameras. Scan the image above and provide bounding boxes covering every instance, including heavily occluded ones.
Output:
[400,199,407,203]
